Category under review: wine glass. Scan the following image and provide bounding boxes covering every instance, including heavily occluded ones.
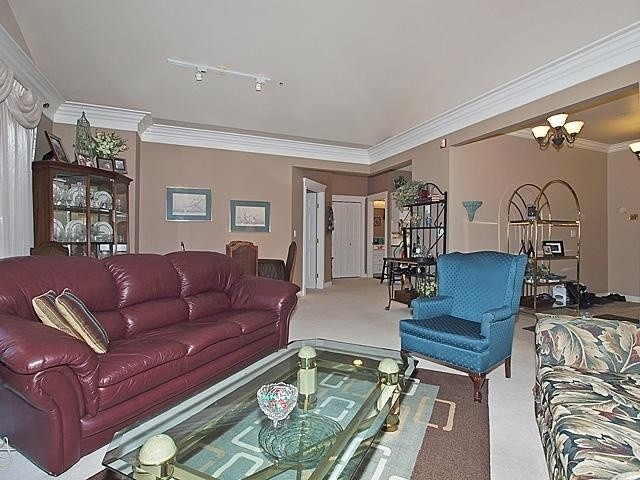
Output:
[53,191,74,211]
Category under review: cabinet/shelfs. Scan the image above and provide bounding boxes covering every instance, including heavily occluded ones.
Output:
[32,161,134,260]
[506,181,580,320]
[384,182,448,311]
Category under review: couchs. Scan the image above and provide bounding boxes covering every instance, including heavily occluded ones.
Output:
[532,313,640,479]
[0,248,299,478]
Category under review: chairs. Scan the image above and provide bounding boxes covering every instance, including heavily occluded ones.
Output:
[258,258,286,281]
[284,241,297,281]
[399,249,529,401]
[30,242,68,255]
[227,240,259,278]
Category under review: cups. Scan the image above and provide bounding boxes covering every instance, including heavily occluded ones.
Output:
[54,224,99,242]
[426,217,433,227]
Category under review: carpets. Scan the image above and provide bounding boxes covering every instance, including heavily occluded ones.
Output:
[89,350,492,479]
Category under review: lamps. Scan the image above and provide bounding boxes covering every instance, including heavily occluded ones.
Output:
[628,142,640,160]
[532,113,585,147]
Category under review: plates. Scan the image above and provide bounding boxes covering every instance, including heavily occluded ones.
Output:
[53,217,113,242]
[52,183,112,211]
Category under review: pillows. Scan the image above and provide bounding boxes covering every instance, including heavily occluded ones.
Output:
[56,289,109,354]
[31,289,84,342]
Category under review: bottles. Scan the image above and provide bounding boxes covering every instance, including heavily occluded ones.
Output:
[412,243,421,258]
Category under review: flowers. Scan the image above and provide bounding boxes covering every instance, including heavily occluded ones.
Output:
[90,128,128,156]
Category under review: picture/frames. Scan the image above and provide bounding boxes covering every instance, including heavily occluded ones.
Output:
[543,240,564,256]
[164,185,271,233]
[76,151,128,174]
[45,130,70,162]
